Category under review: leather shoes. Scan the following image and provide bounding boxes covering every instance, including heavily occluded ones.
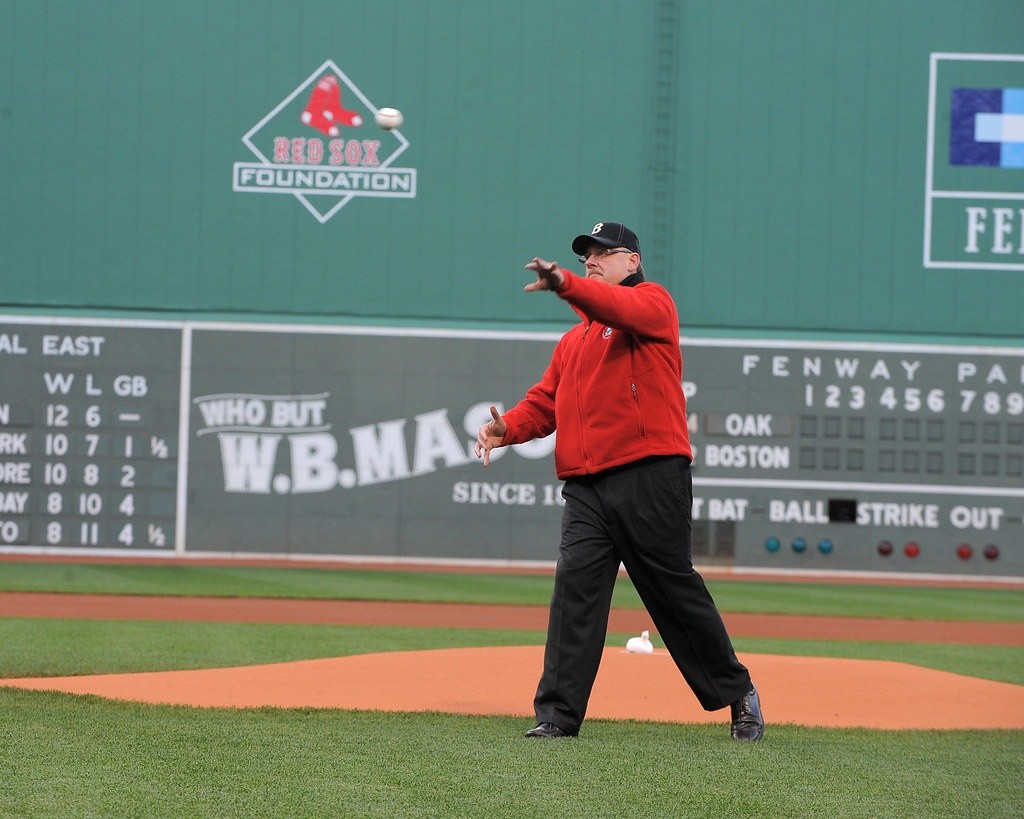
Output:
[524,723,568,738]
[730,682,764,743]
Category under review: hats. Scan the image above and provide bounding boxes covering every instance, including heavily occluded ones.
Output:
[572,223,641,261]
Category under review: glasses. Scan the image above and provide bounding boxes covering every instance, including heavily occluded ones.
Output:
[578,248,633,263]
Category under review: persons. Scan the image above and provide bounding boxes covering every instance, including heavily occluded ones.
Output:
[474,223,764,742]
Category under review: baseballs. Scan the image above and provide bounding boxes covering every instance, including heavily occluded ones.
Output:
[375,107,403,130]
